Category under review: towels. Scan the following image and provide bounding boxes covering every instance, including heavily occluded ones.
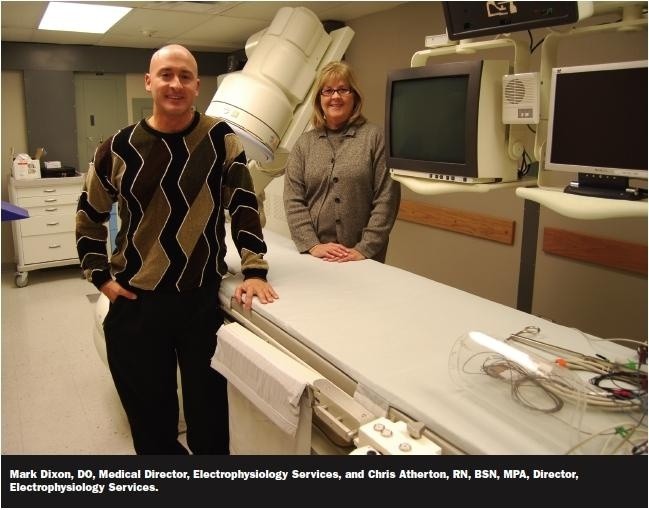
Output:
[209,321,316,455]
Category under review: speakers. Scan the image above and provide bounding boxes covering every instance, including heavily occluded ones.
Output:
[502,72,542,124]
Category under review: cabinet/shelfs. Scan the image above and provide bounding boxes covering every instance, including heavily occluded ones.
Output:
[1,177,87,288]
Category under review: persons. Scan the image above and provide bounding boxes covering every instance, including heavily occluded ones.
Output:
[75,41,279,457]
[283,60,401,264]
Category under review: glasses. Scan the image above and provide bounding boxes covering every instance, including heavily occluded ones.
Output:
[321,86,352,96]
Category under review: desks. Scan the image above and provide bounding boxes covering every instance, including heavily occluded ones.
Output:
[217,222,647,455]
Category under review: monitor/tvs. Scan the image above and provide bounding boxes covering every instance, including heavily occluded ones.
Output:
[544,59,648,201]
[384,59,515,184]
[441,1,594,41]
